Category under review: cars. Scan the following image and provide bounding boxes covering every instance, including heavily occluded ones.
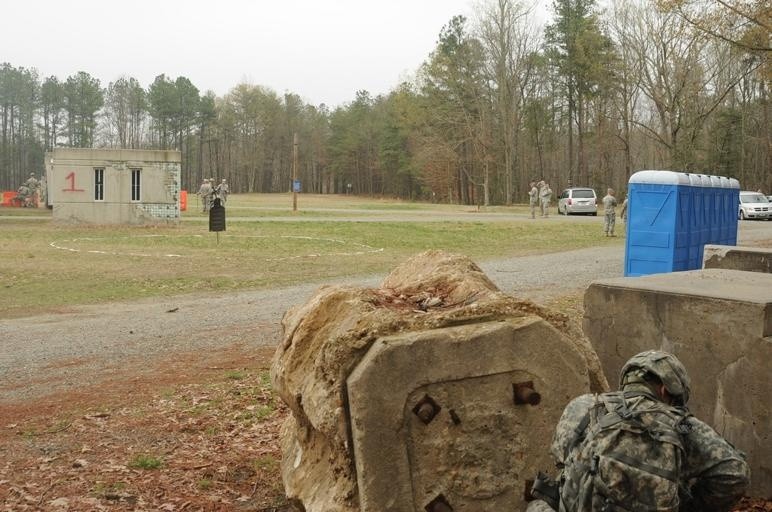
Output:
[556,187,598,216]
[738,191,772,221]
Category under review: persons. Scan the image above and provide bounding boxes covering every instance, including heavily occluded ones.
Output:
[526,350,752,511]
[196,177,230,212]
[528,180,552,220]
[620,193,628,225]
[602,188,618,237]
[16,173,45,208]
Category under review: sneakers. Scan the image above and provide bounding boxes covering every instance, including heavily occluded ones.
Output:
[605,232,618,238]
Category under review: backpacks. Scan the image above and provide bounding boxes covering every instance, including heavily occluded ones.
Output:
[557,390,693,512]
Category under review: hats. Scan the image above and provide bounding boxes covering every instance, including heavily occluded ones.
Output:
[607,188,616,194]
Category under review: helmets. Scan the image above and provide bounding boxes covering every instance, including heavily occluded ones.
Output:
[617,349,692,407]
